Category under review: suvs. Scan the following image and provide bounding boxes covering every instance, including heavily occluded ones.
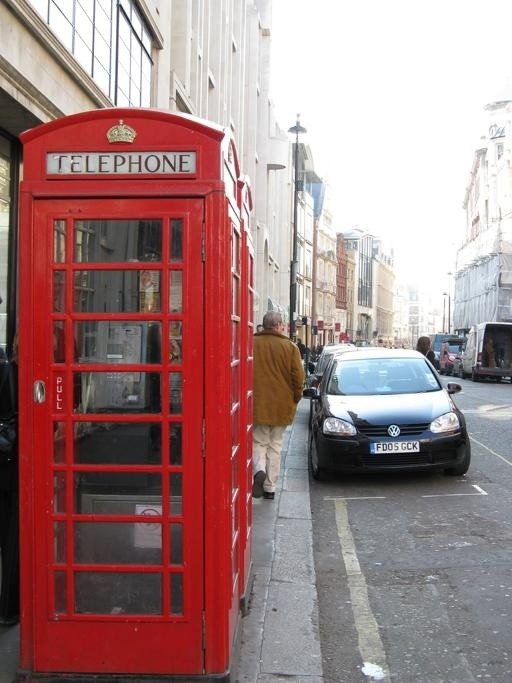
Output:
[440,338,464,376]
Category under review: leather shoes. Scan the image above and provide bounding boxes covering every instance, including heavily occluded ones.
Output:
[252,470,266,498]
[264,492,275,499]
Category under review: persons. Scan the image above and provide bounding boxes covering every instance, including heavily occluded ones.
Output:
[485,338,498,368]
[416,336,440,375]
[251,312,304,498]
[294,336,307,360]
[256,324,265,332]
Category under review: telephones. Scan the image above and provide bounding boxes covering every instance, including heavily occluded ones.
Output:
[78,320,148,410]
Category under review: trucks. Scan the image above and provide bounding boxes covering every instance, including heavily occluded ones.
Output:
[463,321,512,383]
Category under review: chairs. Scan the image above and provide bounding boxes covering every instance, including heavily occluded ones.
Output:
[339,365,415,394]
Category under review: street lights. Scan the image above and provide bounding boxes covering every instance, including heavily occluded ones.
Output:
[443,292,451,334]
[288,113,307,341]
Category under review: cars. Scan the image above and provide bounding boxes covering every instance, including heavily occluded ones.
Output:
[302,343,471,482]
